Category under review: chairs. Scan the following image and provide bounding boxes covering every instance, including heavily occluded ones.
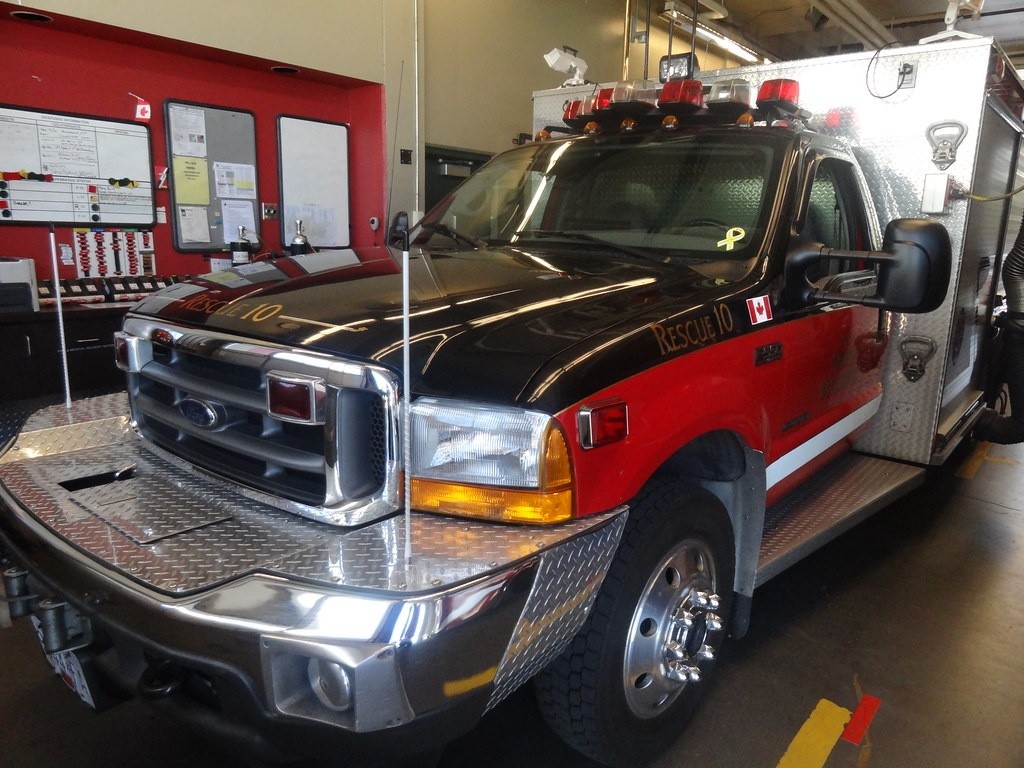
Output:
[605,201,647,228]
[756,209,816,239]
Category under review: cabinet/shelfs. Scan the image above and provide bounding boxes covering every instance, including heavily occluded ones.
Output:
[0,301,138,404]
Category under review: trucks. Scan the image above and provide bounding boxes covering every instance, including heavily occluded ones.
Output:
[0,31,1023,768]
